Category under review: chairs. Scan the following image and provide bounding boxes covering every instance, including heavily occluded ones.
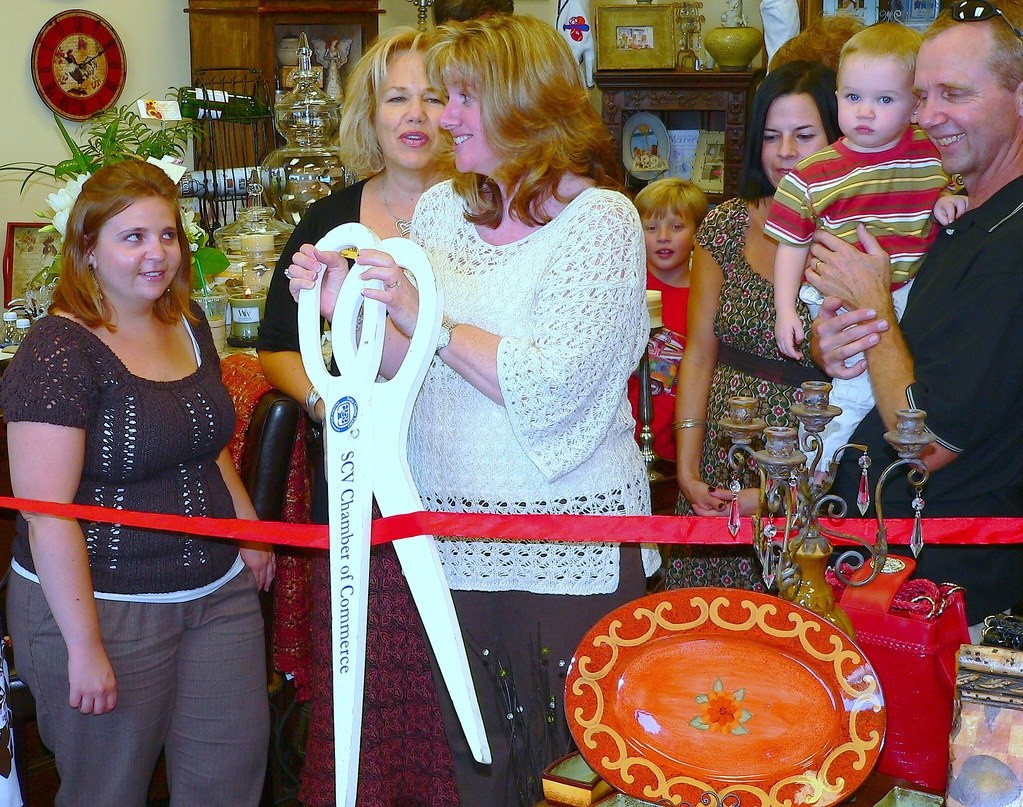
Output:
[0,388,302,807]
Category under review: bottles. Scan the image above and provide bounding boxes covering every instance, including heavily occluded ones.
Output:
[277,34,303,65]
[212,183,296,288]
[0,311,32,351]
[177,86,274,125]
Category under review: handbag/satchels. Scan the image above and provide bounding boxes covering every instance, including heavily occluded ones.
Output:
[822,554,973,795]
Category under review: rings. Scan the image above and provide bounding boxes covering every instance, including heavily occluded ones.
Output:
[815,261,822,272]
[386,278,400,287]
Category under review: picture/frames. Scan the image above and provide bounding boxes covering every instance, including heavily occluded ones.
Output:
[595,4,676,69]
[689,129,725,194]
[905,0,939,22]
[822,0,880,27]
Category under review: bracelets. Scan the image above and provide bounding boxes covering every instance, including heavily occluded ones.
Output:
[672,418,706,431]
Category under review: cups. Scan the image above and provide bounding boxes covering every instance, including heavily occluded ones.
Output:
[190,292,229,355]
[227,285,268,347]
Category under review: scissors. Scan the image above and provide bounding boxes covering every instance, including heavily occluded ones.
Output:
[296,221,494,800]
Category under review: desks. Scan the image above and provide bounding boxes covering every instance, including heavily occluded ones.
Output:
[594,67,766,207]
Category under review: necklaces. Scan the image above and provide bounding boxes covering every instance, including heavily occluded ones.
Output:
[382,175,411,238]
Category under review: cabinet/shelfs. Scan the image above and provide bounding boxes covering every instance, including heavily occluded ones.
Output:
[184,0,388,222]
[188,66,279,250]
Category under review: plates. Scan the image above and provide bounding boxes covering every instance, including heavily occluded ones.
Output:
[562,585,887,807]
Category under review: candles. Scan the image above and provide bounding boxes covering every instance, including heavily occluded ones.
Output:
[646,289,663,328]
[230,288,267,338]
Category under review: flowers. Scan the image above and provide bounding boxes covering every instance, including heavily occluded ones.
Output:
[0,87,232,293]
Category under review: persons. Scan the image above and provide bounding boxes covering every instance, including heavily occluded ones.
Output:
[628,178,710,463]
[255,25,447,807]
[809,0,1023,647]
[665,18,866,596]
[0,161,278,807]
[287,14,663,807]
[764,22,969,486]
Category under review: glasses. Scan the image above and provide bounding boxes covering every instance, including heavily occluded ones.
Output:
[935,0,1023,43]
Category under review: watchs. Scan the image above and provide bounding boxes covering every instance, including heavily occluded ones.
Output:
[435,320,459,355]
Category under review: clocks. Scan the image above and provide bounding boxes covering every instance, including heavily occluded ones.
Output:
[32,10,127,122]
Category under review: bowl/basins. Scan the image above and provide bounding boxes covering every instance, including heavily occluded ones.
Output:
[703,26,764,72]
